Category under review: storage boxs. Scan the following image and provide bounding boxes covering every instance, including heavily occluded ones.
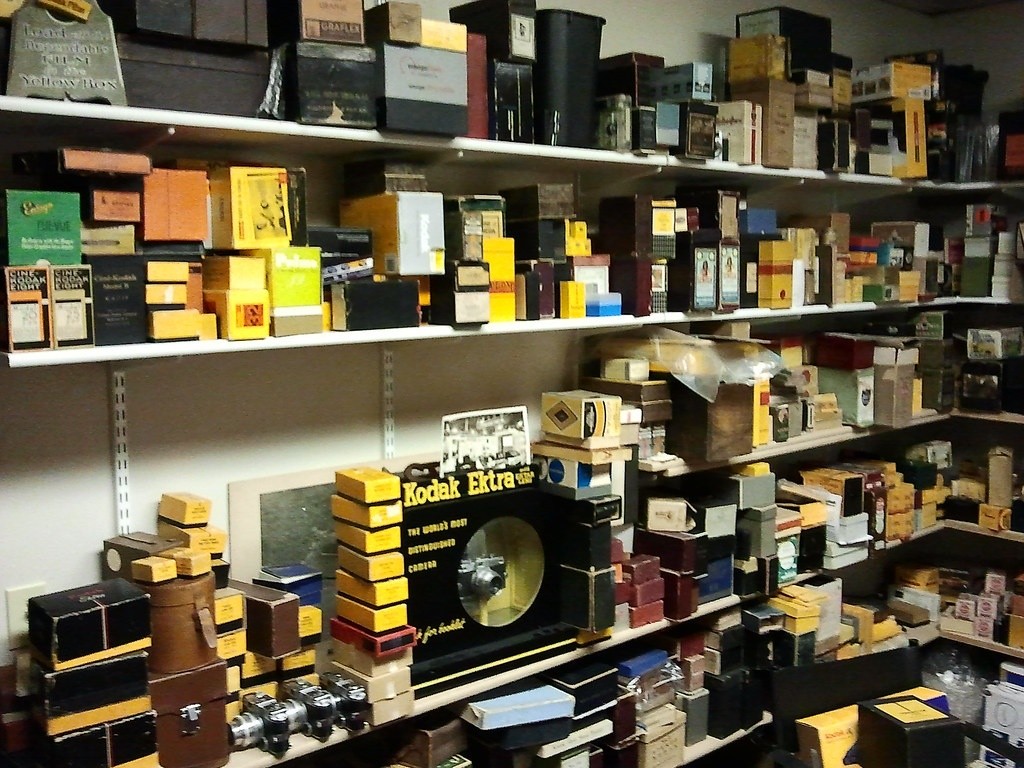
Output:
[0,0,1024,182]
[0,307,1024,768]
[0,148,1024,352]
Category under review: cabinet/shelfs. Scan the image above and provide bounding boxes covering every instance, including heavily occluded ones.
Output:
[0,93,1024,768]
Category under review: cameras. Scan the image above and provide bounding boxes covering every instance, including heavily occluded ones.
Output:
[227,671,369,759]
[455,553,508,601]
